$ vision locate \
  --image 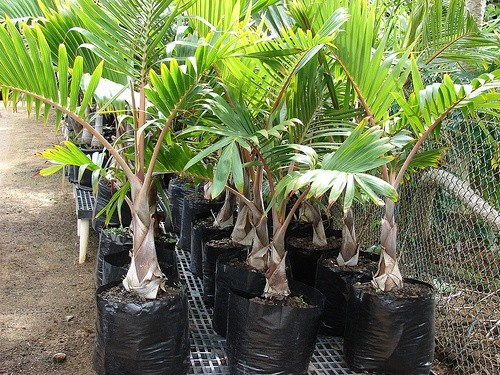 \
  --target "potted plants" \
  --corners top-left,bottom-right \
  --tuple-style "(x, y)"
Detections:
(0, 1), (500, 375)
